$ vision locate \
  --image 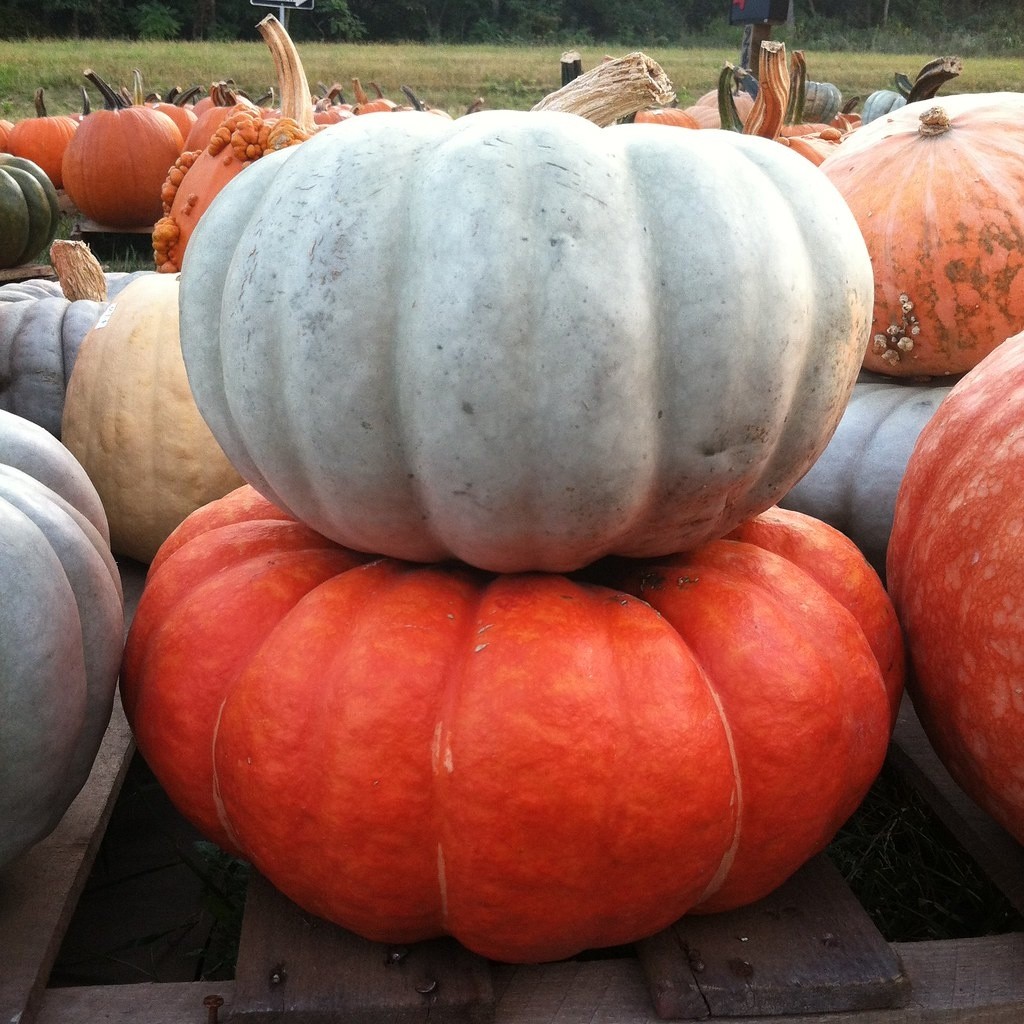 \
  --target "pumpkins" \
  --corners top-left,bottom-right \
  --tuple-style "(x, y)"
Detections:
(0, 4), (1023, 962)
